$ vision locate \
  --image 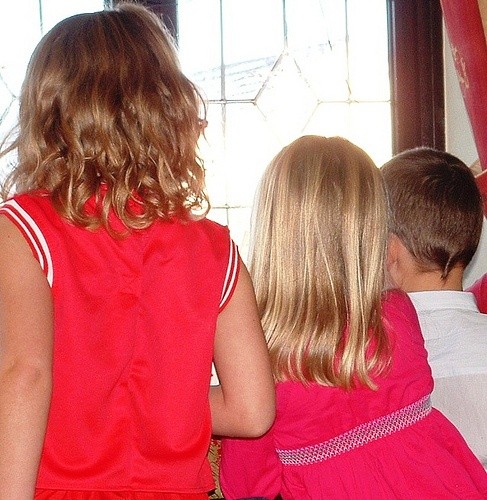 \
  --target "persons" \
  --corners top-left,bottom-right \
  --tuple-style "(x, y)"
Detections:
(379, 147), (487, 474)
(219, 135), (487, 500)
(0, 0), (276, 500)
